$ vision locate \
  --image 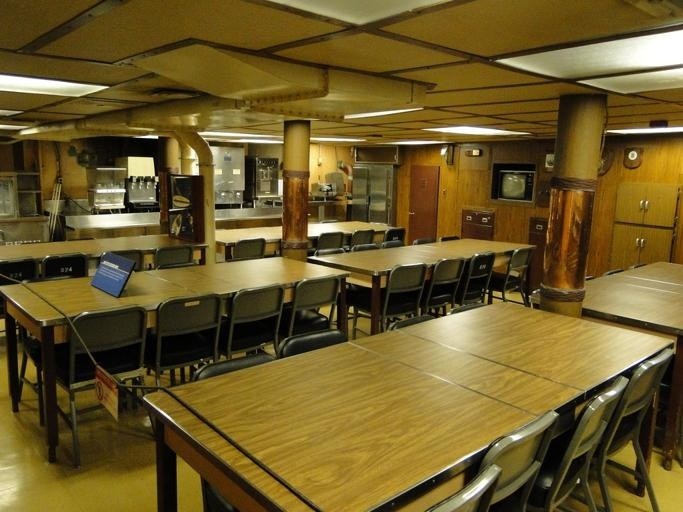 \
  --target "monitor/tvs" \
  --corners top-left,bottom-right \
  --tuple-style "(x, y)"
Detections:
(498, 170), (535, 202)
